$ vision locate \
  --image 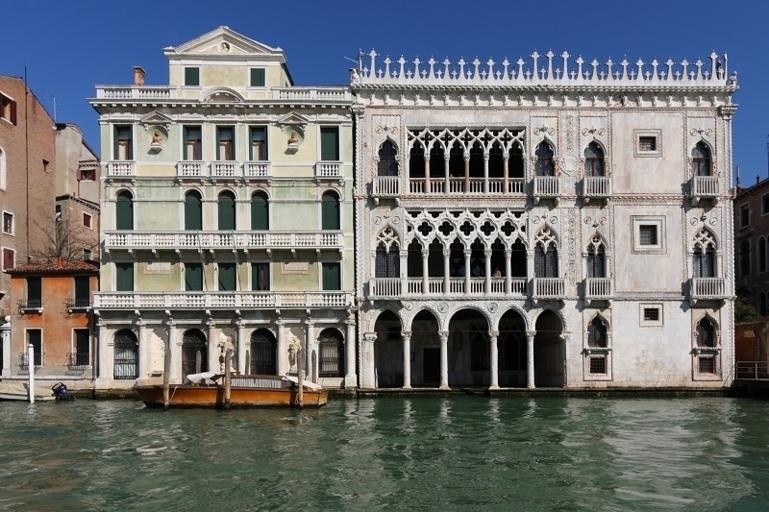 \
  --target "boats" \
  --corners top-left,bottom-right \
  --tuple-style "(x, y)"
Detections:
(0, 383), (75, 401)
(133, 374), (329, 408)
